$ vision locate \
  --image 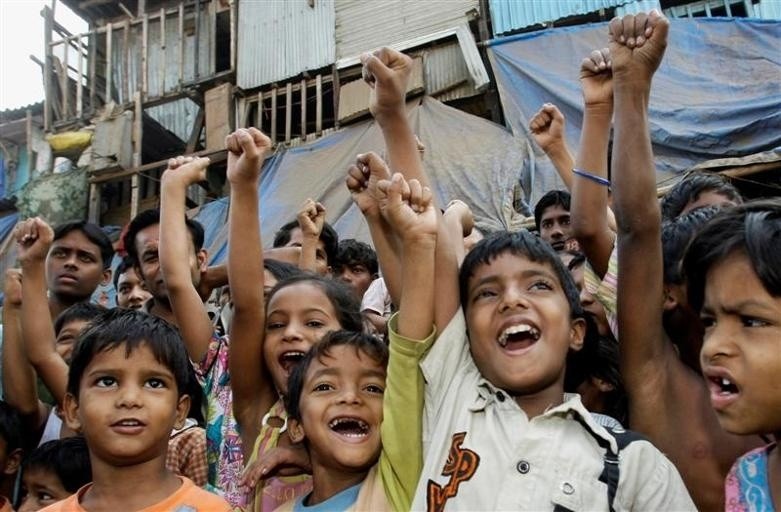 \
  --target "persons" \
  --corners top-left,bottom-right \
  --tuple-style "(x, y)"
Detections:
(0, 7), (779, 510)
